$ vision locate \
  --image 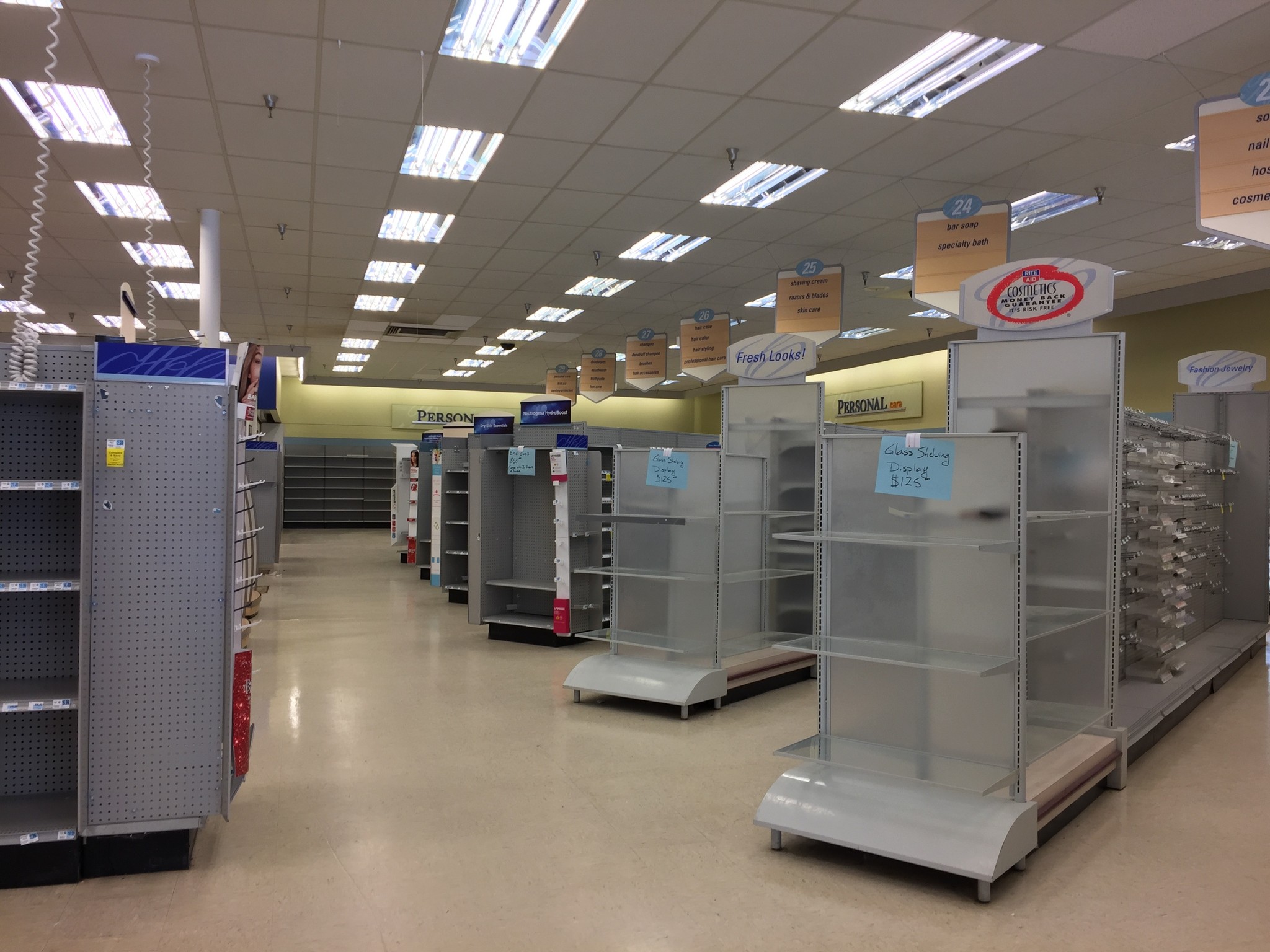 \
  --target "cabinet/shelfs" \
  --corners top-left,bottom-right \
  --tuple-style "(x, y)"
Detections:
(0, 251), (1270, 911)
